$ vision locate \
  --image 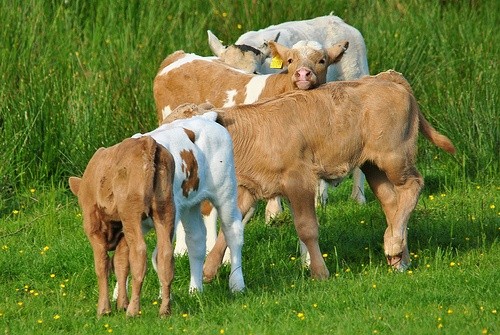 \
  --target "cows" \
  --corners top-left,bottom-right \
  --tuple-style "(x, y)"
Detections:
(158, 69), (457, 286)
(112, 110), (246, 302)
(68, 134), (176, 318)
(207, 14), (371, 228)
(154, 39), (351, 270)
